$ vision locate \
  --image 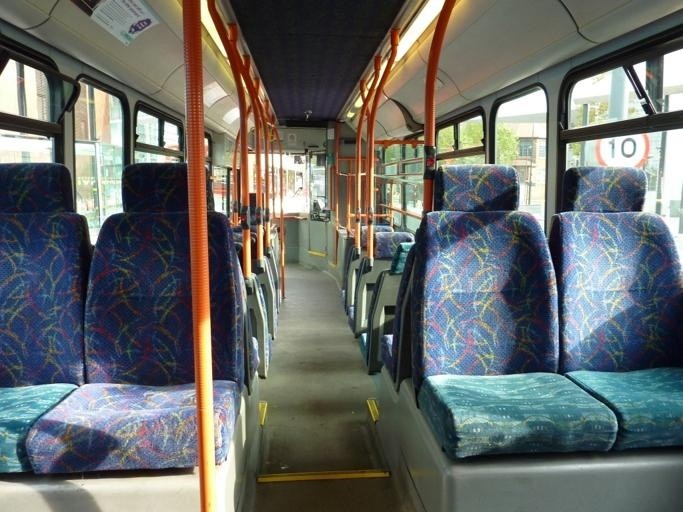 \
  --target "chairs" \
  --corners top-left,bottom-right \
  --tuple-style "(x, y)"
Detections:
(342, 225), (412, 377)
(383, 163), (683, 463)
(2, 159), (243, 476)
(231, 215), (282, 380)
